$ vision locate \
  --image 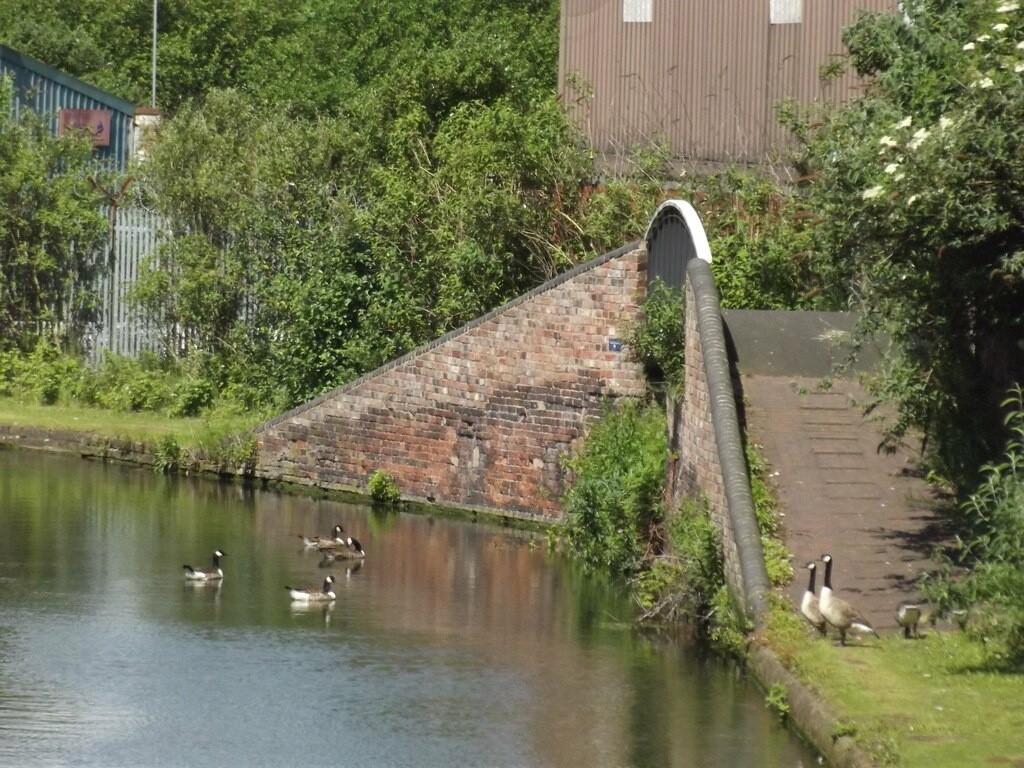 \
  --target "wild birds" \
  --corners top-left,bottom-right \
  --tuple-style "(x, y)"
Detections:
(298, 525), (346, 549)
(318, 537), (366, 560)
(799, 561), (840, 640)
(815, 554), (880, 647)
(285, 576), (338, 601)
(894, 599), (922, 639)
(182, 549), (225, 580)
